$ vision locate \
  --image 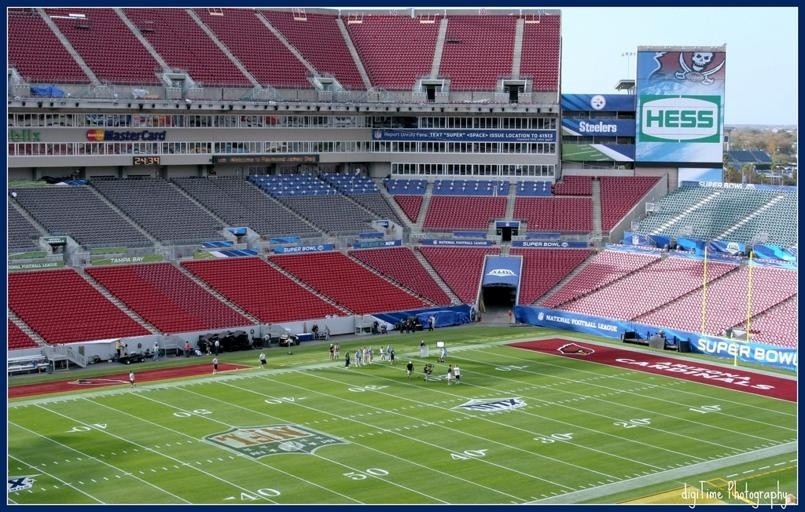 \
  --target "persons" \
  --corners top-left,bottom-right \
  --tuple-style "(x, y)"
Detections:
(116, 340), (127, 359)
(448, 365), (461, 385)
(374, 321), (379, 333)
(324, 327), (329, 341)
(330, 343), (340, 360)
(213, 358), (218, 375)
(407, 361), (414, 378)
(130, 370), (135, 388)
(215, 341), (219, 355)
(204, 338), (212, 355)
(184, 341), (191, 357)
(312, 322), (319, 340)
(420, 340), (427, 355)
(424, 365), (432, 383)
(344, 345), (394, 368)
(400, 316), (435, 333)
(259, 352), (266, 368)
(438, 348), (447, 362)
(153, 344), (159, 361)
